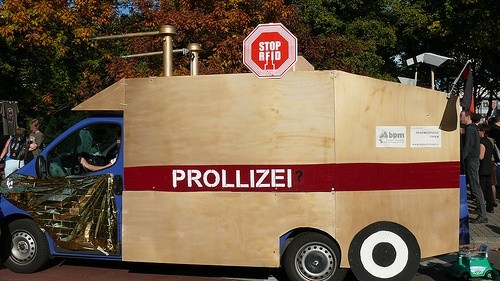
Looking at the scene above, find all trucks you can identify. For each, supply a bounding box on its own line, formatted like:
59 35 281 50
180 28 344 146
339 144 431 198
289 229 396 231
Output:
0 69 459 281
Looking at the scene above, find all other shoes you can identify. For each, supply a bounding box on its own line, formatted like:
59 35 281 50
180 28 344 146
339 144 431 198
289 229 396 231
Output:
469 200 498 214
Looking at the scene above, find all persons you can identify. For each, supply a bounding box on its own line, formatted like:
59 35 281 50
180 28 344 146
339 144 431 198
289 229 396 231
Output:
474 101 500 202
80 137 121 171
460 110 488 224
0 127 26 178
477 124 495 213
26 119 45 163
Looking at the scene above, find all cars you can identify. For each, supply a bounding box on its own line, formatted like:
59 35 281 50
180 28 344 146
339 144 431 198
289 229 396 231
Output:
447 245 496 281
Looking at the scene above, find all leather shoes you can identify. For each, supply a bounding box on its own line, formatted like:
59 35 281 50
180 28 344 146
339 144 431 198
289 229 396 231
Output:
469 214 489 224
